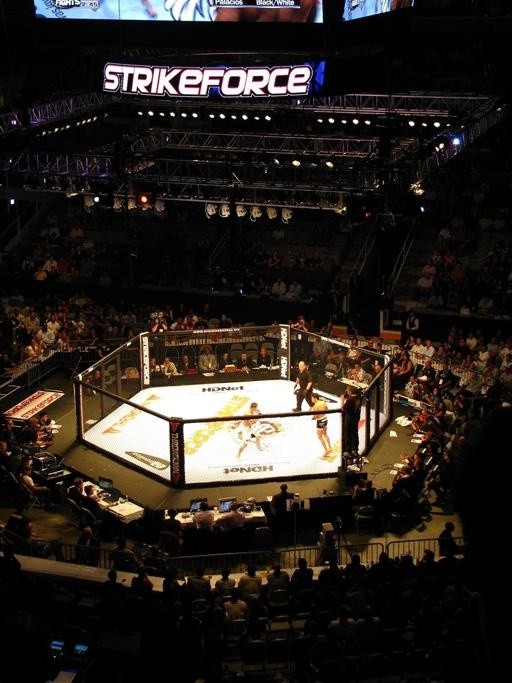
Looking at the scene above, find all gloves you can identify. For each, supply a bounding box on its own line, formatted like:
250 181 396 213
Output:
302 388 308 397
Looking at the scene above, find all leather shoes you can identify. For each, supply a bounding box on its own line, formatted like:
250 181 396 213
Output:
293 407 301 411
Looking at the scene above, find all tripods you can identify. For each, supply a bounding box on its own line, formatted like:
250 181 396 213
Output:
316 528 352 565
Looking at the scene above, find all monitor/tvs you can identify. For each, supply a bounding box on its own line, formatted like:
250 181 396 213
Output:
217 497 237 512
99 477 113 491
189 497 207 512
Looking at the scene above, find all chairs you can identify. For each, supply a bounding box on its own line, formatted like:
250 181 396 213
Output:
126 343 282 366
0 447 509 683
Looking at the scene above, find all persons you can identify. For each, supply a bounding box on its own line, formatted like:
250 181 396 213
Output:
2 0 511 681
